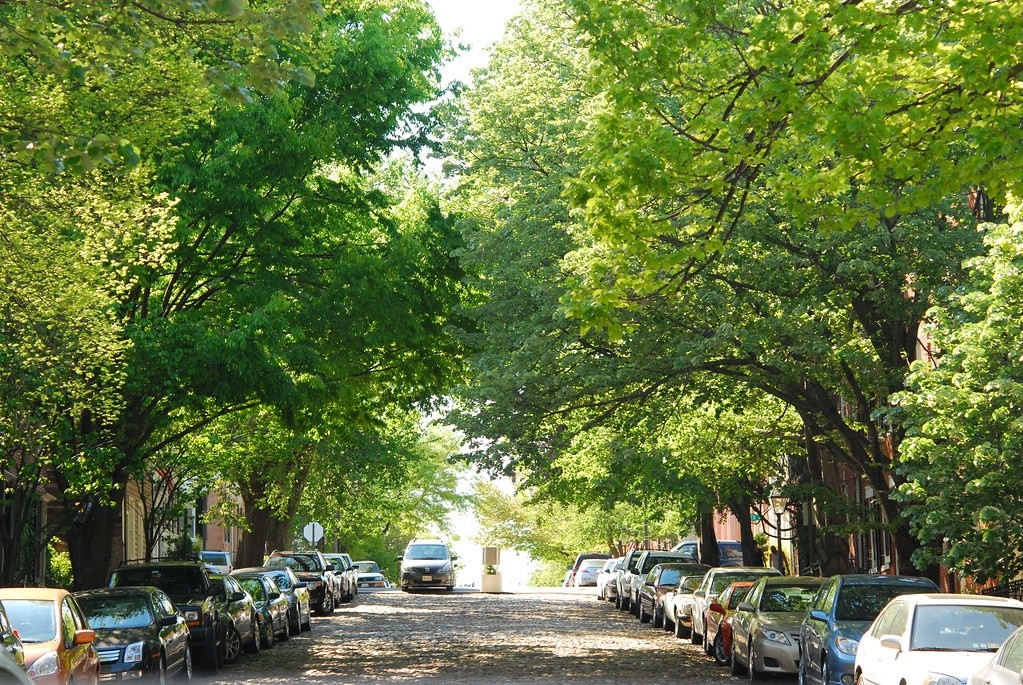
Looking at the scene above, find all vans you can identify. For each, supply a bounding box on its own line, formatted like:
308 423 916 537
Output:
670 540 746 566
200 550 234 576
396 539 457 591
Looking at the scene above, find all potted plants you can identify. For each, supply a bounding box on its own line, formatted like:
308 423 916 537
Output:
481 564 503 591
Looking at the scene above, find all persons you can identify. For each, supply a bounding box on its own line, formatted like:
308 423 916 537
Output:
753 540 767 568
769 546 790 576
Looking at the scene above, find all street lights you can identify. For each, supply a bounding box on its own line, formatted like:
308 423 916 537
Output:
63 488 95 591
770 487 788 575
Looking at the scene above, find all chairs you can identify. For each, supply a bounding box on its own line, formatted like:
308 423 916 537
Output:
412 547 425 556
367 567 374 572
965 623 984 636
788 595 803 606
434 548 445 555
919 617 942 643
767 590 786 605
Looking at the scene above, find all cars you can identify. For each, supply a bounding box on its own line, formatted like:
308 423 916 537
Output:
562 553 612 587
322 553 361 608
207 565 311 654
797 574 943 685
691 567 782 644
854 592 1023 685
596 556 625 603
730 575 830 685
354 561 393 588
705 580 756 665
636 561 712 638
0 587 193 685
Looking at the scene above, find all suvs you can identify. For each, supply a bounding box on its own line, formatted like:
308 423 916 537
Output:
107 556 221 674
262 549 335 615
616 548 672 611
